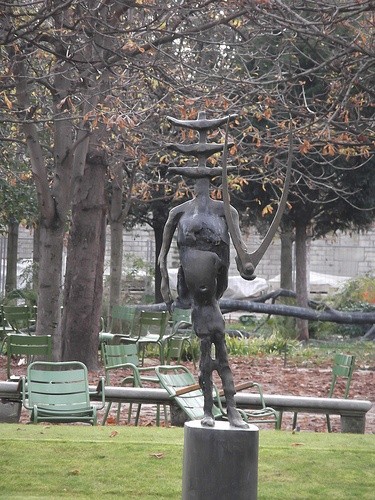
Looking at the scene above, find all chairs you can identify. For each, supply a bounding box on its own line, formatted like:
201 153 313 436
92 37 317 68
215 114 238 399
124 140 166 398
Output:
116 309 168 367
5 333 56 385
161 306 197 366
1 304 37 359
154 362 279 432
97 302 135 363
100 343 168 429
288 352 356 432
25 361 97 426
